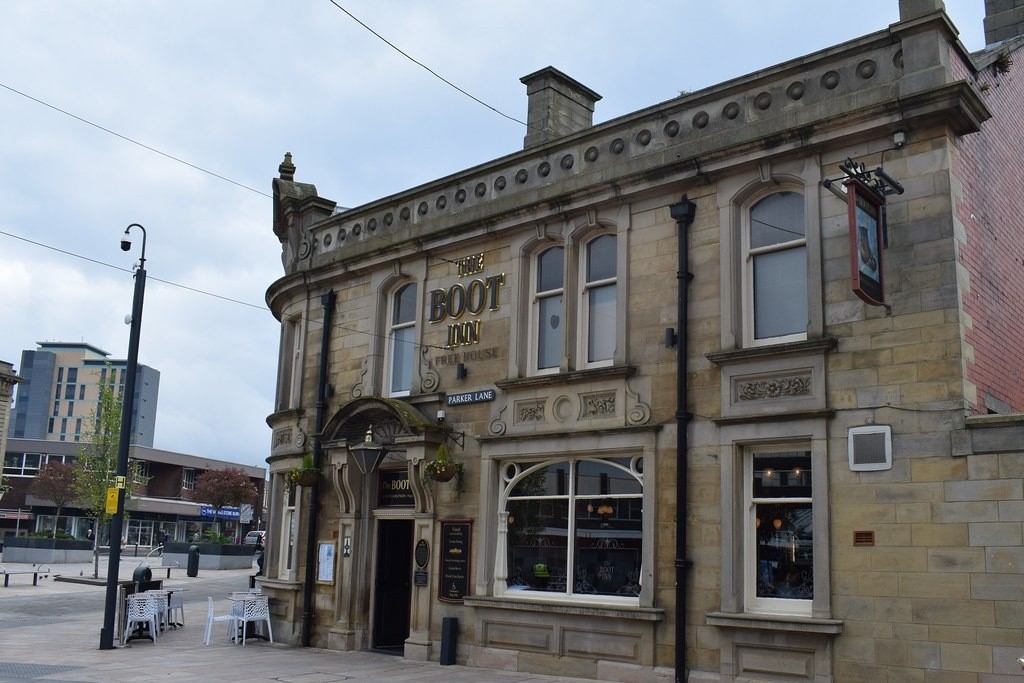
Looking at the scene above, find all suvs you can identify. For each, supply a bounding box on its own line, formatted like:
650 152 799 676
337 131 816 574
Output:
243 531 266 545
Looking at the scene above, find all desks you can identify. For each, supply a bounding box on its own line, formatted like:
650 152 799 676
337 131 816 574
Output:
226 596 270 644
162 588 189 626
125 597 163 642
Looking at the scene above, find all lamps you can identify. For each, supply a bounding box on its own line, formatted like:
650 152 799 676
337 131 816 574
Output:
324 384 334 397
456 363 467 379
665 328 674 348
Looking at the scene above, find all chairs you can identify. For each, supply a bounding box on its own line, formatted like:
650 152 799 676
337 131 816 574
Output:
204 592 273 646
123 590 185 644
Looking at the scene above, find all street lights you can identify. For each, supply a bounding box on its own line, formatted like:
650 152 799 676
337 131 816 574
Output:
97 223 146 647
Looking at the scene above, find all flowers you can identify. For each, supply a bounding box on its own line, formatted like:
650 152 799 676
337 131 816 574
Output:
421 459 466 502
289 455 312 477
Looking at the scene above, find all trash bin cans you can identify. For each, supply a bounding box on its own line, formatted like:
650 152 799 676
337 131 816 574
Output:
0 543 3 553
230 537 234 542
165 534 174 542
187 545 200 577
133 567 152 583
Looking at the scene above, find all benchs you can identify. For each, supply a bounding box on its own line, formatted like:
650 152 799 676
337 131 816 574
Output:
0 564 50 587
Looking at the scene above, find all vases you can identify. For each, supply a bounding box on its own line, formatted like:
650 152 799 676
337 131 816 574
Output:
432 465 456 482
295 472 315 487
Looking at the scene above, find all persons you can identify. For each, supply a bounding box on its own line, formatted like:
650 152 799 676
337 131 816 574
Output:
156 528 165 547
86 529 95 540
193 531 200 542
